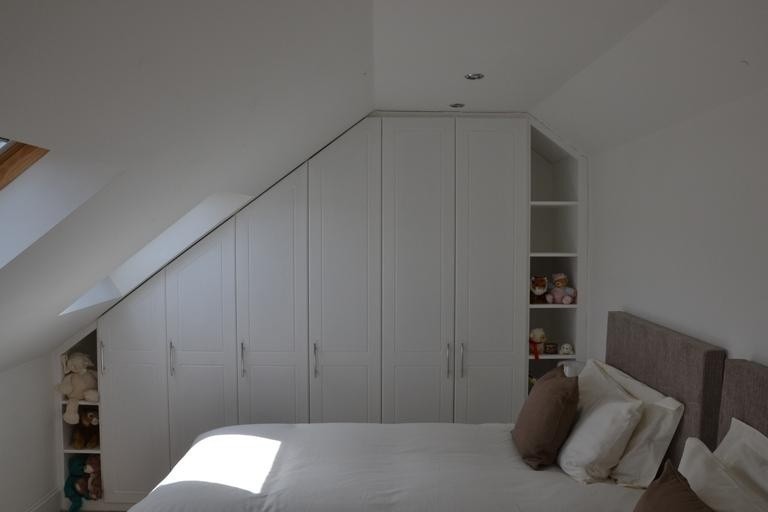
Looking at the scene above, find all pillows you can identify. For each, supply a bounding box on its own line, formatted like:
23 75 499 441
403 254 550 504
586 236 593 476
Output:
504 362 584 473
628 470 710 510
554 358 646 485
675 433 763 510
589 356 688 489
710 414 765 496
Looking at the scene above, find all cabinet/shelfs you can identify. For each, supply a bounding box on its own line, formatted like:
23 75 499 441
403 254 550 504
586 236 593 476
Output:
54 108 588 501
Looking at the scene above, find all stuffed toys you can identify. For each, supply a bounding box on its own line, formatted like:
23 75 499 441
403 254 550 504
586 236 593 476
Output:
530 324 547 356
558 340 574 355
60 351 103 508
531 270 574 304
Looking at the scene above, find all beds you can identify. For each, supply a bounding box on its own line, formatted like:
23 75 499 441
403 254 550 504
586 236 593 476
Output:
126 313 729 510
